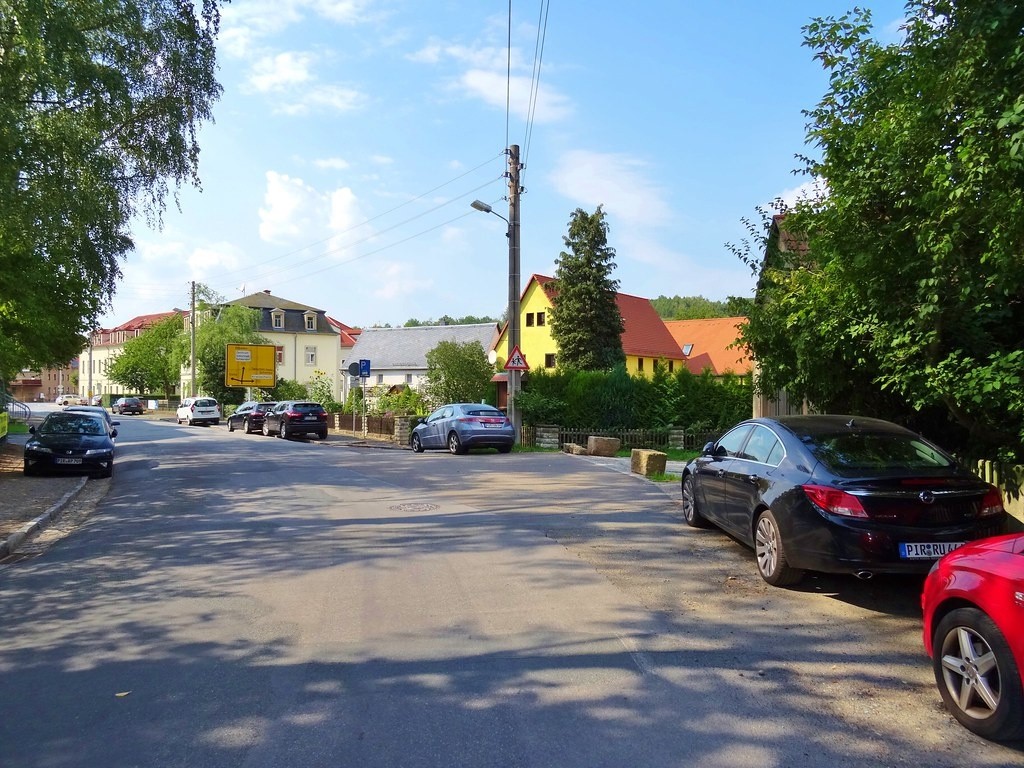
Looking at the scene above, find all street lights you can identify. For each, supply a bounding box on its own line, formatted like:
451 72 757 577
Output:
173 307 195 397
470 200 520 444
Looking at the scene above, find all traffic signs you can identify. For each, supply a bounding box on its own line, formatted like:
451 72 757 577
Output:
225 344 276 387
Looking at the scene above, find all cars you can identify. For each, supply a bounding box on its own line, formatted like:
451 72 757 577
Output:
24 412 117 476
63 406 121 443
112 398 144 415
176 397 221 426
682 414 1009 586
227 400 277 434
411 403 515 455
921 532 1024 741
56 395 101 406
262 401 328 438
